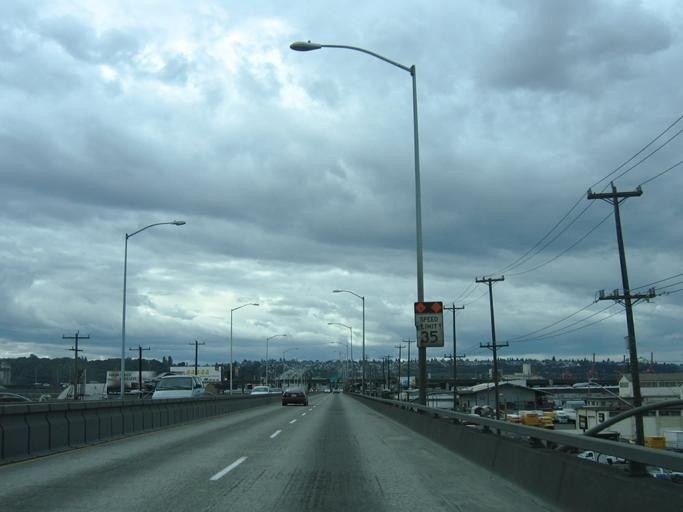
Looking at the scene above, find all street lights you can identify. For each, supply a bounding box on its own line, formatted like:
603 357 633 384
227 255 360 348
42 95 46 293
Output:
265 334 288 387
328 320 355 390
288 39 426 406
119 220 187 398
333 288 365 395
230 303 261 395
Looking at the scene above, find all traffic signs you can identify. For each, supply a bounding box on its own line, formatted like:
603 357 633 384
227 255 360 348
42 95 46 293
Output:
413 300 444 349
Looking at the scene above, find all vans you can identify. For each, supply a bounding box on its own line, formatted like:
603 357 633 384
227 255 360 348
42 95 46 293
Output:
151 374 207 399
281 388 309 406
249 386 273 394
